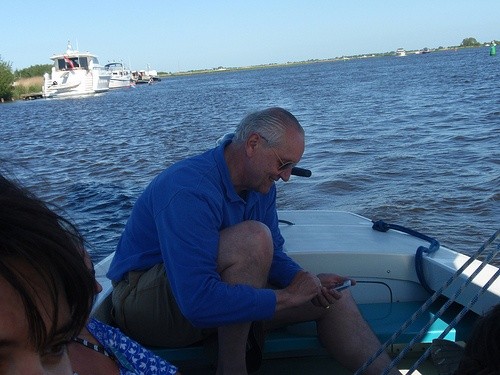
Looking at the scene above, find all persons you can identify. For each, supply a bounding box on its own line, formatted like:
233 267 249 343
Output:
0 167 179 375
106 108 403 375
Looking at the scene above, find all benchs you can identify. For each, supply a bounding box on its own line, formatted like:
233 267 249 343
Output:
152 301 473 361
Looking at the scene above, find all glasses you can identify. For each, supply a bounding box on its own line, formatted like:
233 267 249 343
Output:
258 134 297 171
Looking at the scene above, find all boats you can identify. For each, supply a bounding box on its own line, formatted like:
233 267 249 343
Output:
41 40 112 100
104 58 162 89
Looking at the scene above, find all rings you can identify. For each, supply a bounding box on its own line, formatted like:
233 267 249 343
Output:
325 305 329 309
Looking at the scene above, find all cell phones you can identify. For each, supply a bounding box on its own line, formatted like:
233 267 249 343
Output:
321 280 351 295
